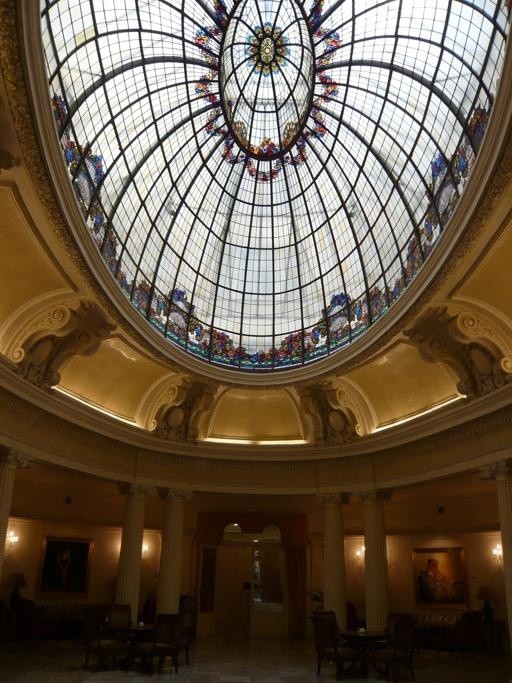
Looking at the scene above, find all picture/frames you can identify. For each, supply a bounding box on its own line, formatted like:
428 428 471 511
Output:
409 545 471 610
36 535 93 599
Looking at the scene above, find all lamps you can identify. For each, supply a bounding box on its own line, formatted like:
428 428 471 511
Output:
6 571 27 607
476 585 498 621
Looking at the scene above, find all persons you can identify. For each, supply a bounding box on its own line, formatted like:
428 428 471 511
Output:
419 557 448 601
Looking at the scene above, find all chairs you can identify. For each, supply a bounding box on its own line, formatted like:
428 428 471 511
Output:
79 601 197 674
310 609 418 681
8 594 62 657
433 611 484 664
